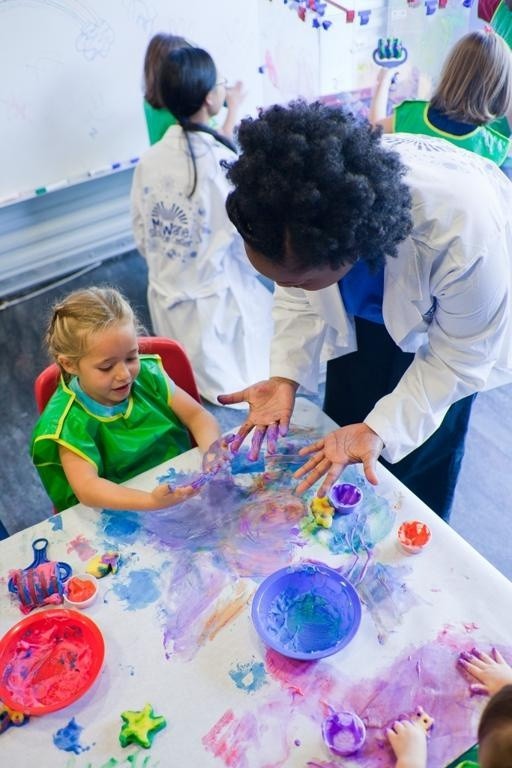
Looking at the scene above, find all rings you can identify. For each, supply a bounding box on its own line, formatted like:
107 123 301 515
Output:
266 420 279 426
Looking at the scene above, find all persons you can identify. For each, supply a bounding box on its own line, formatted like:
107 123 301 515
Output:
141 32 250 151
385 643 512 768
213 97 512 533
369 27 512 170
30 286 238 516
130 46 274 411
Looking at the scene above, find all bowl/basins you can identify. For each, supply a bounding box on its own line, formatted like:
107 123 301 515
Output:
252 564 362 663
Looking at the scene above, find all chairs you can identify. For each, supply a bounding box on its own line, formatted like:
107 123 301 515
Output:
35 338 203 449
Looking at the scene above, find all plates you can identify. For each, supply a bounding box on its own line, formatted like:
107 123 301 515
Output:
0 607 106 719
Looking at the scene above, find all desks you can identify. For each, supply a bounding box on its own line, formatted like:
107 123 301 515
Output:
0 397 512 768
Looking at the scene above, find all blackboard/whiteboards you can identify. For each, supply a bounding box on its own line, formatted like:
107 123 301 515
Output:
2 0 478 208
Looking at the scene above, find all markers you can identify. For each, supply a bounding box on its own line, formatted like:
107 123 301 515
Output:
33 178 70 195
89 162 121 177
128 156 143 165
